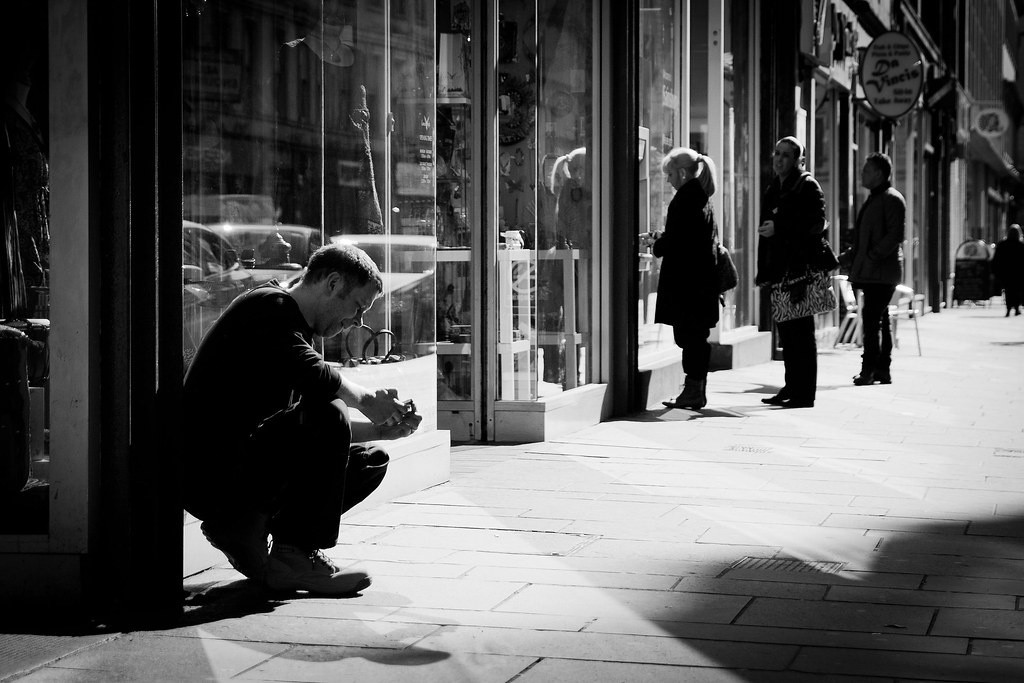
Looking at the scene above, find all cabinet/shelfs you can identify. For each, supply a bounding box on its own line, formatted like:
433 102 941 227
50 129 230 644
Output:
400 248 531 402
530 250 593 392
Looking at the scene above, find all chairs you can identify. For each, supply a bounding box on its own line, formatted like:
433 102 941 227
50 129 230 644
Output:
858 283 921 356
831 274 902 348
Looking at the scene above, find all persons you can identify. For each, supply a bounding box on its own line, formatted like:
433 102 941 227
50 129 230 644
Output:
754 136 840 409
182 243 424 594
991 224 1024 317
837 153 906 385
550 147 592 391
639 147 738 411
279 0 384 234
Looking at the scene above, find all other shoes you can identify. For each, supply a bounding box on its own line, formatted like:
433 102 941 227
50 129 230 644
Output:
762 391 818 408
854 369 893 385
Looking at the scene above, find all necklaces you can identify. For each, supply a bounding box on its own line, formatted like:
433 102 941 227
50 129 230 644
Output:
308 33 341 63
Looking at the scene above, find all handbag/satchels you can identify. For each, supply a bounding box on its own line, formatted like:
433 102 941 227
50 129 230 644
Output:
771 264 838 324
716 244 741 294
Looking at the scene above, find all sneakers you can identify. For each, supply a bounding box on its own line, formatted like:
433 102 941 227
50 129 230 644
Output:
265 534 372 597
201 519 273 584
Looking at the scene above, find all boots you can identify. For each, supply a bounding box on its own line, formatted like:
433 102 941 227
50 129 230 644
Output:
662 374 710 412
